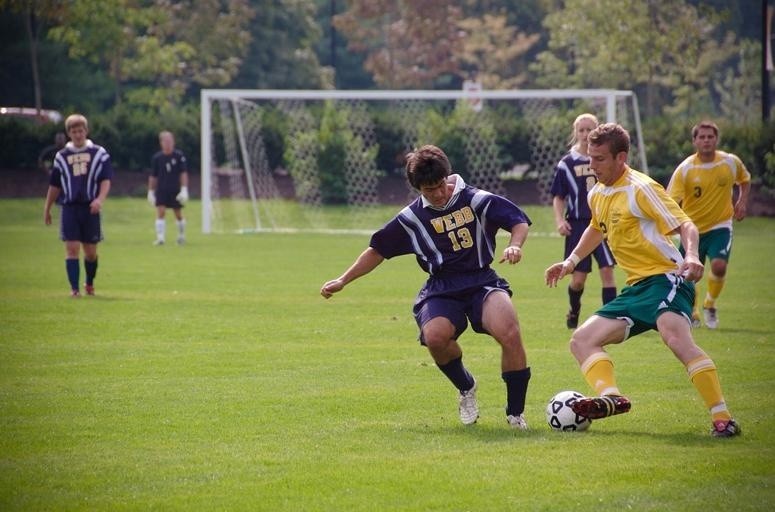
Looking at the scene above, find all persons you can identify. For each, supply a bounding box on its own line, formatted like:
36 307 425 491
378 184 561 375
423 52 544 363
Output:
314 140 535 433
665 121 755 330
553 111 618 329
545 121 742 437
38 130 67 175
40 111 114 301
142 128 192 249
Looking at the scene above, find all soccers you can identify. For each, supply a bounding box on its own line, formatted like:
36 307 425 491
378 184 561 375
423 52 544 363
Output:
545 391 592 433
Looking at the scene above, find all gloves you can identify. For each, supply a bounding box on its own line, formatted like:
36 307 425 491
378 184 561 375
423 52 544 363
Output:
148 190 157 207
176 186 189 205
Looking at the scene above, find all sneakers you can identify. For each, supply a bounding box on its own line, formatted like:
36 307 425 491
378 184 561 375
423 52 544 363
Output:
713 418 741 437
703 308 718 329
571 395 631 419
458 375 479 425
567 307 580 329
505 404 531 433
84 284 94 295
691 319 701 328
72 289 80 296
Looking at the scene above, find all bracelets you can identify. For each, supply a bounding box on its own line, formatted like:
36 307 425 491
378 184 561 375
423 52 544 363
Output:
564 252 584 266
